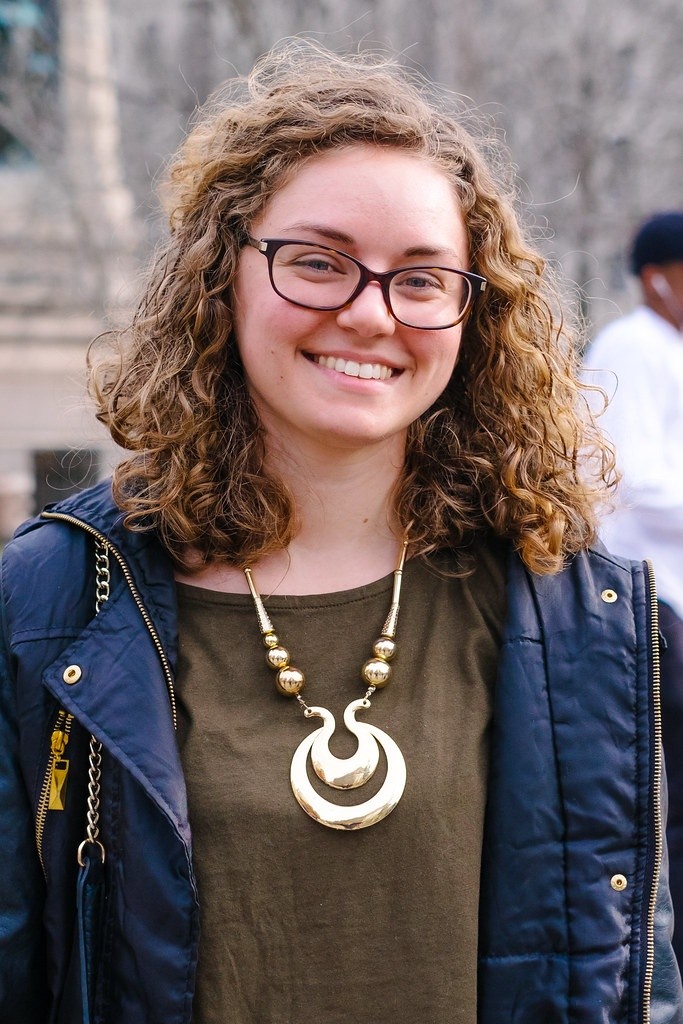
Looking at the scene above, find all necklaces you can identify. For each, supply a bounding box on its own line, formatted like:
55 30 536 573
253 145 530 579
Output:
233 501 437 834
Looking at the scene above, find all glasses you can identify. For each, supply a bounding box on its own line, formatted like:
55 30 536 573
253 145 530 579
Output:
231 233 493 332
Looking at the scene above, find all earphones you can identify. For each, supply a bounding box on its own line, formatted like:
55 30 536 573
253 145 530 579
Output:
650 274 671 297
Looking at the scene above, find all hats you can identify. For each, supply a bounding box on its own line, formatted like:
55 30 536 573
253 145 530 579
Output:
630 211 683 274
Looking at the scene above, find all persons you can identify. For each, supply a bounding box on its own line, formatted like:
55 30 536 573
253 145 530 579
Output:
567 206 683 987
0 45 683 1024
